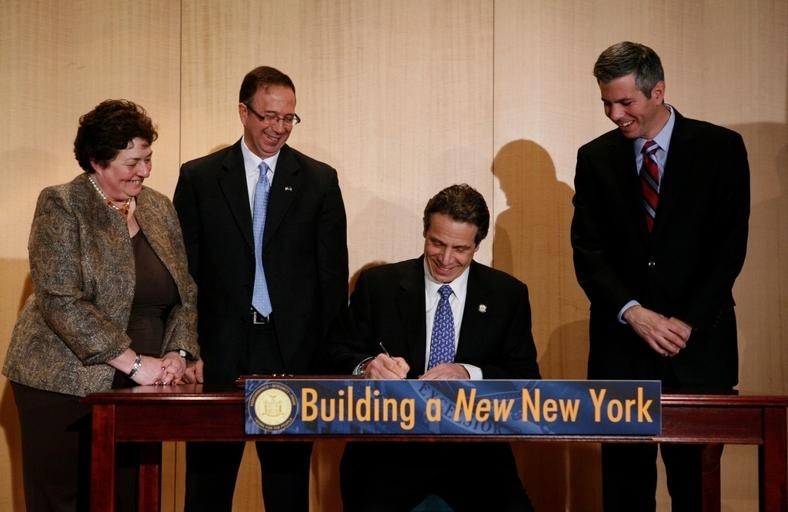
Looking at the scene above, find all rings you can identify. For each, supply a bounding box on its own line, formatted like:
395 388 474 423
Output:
379 342 392 358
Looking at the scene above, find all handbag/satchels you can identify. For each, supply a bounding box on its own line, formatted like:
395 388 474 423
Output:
357 358 373 377
128 355 142 379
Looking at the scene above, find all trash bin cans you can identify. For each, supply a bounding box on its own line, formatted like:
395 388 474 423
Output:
173 349 190 362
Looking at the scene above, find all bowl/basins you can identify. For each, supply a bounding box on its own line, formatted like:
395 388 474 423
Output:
87 176 132 220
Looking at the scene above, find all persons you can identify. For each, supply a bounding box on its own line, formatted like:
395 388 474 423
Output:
173 66 349 511
571 41 750 511
340 185 541 511
2 99 197 512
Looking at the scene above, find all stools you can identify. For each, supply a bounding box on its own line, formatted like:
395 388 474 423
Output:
250 310 275 328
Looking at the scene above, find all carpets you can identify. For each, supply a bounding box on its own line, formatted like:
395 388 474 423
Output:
428 283 456 371
639 141 662 231
249 161 277 318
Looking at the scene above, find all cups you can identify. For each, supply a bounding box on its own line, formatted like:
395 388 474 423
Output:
243 102 301 127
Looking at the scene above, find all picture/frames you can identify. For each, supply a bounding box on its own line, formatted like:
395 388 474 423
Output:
87 391 788 512
